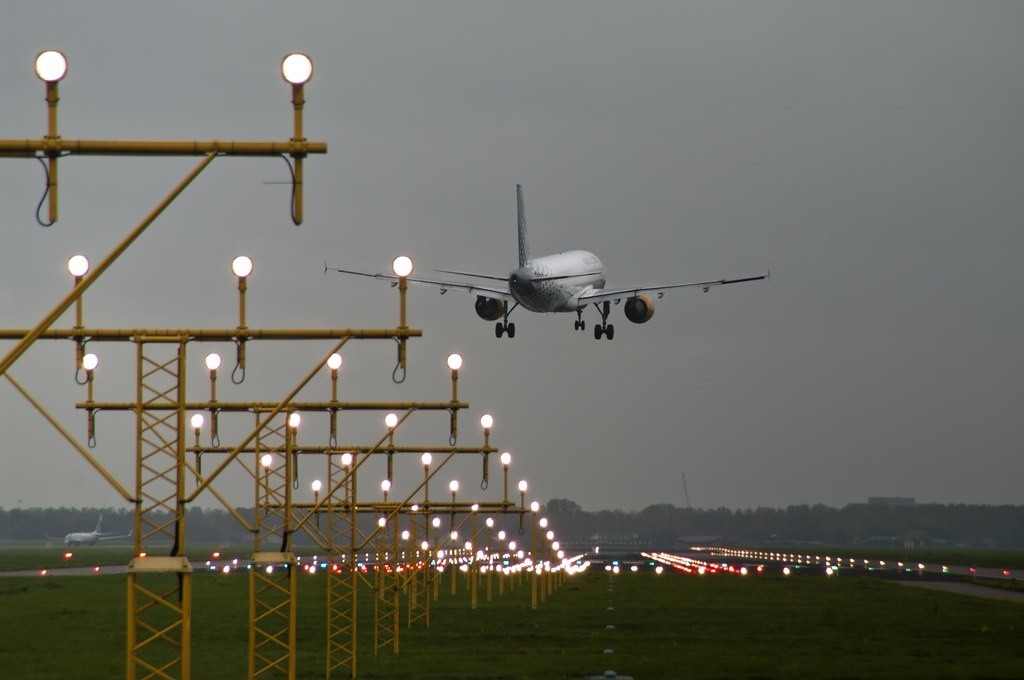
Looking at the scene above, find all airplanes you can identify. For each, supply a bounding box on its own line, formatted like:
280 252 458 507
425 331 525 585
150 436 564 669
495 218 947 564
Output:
339 183 768 340
48 514 135 547
